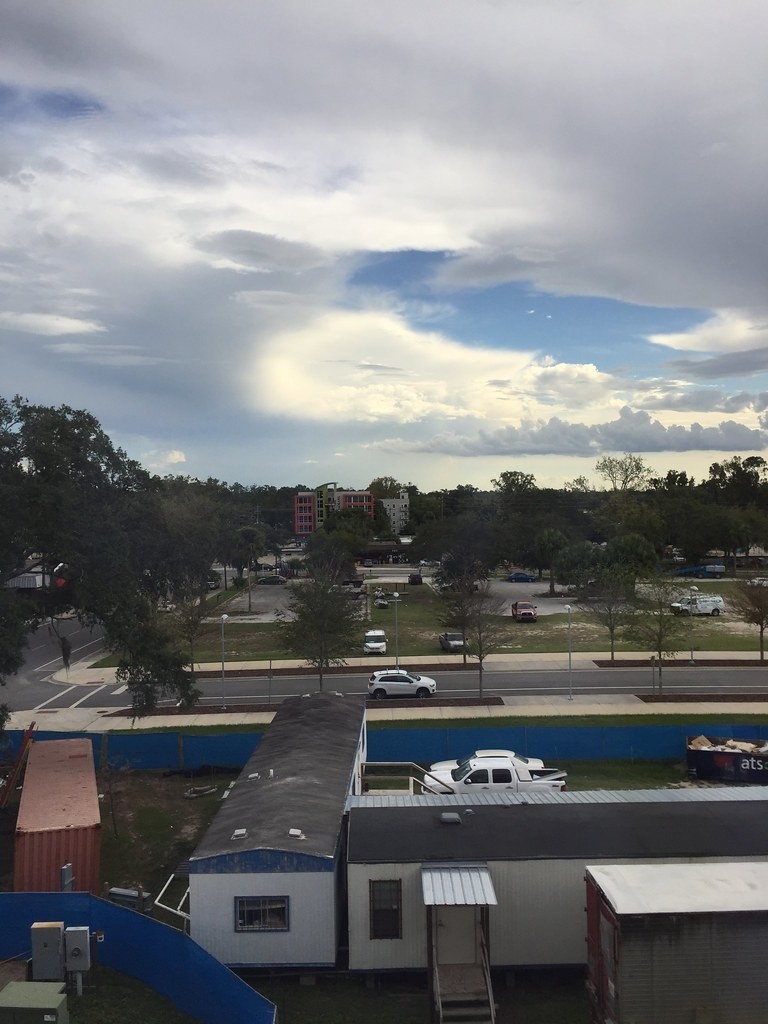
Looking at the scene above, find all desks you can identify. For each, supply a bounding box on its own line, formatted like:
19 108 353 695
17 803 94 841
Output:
386 591 410 601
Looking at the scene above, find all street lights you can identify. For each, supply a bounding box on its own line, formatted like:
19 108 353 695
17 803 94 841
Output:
393 592 400 670
690 586 698 664
564 605 574 700
221 614 228 709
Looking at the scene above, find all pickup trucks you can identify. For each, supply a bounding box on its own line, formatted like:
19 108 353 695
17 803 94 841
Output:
512 601 537 623
440 582 479 593
206 577 220 589
420 758 568 795
439 632 469 653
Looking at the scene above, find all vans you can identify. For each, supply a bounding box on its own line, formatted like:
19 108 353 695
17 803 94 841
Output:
670 593 726 617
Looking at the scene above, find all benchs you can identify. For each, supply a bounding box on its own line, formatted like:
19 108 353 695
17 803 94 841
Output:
387 600 402 603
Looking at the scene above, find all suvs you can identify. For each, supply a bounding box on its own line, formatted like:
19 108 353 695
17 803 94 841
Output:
368 670 436 698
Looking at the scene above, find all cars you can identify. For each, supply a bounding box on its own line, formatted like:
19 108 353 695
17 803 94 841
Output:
252 563 273 571
673 549 686 555
408 574 422 584
374 599 389 608
747 577 768 588
429 750 545 772
673 555 686 563
363 629 388 655
157 601 177 613
258 575 287 585
508 572 535 582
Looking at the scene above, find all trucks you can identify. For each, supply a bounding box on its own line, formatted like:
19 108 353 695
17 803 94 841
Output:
313 569 366 588
694 563 725 579
